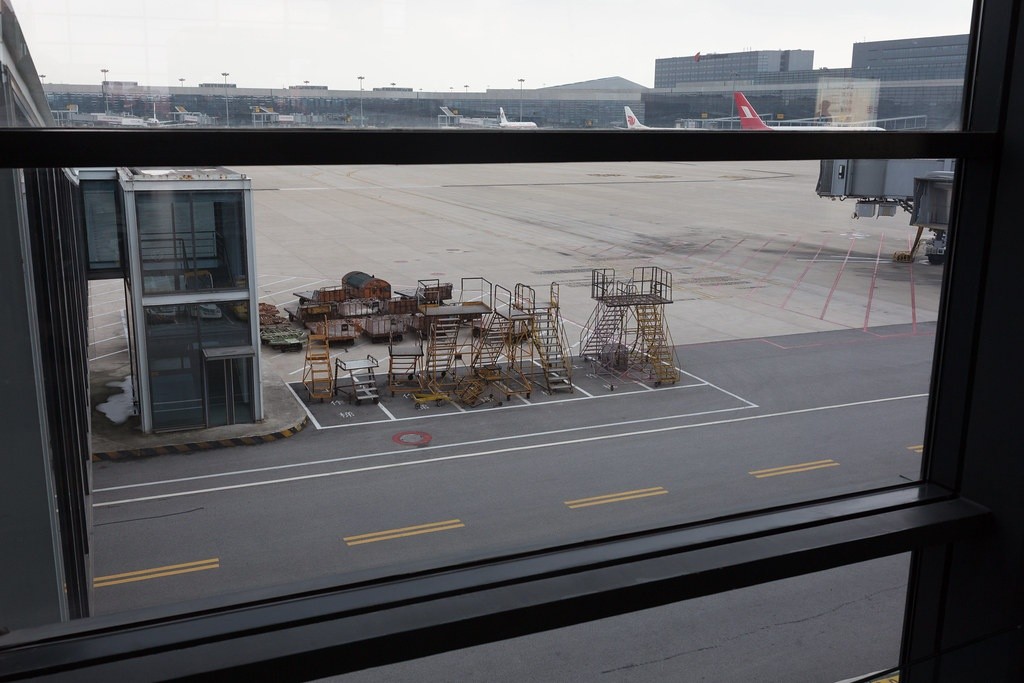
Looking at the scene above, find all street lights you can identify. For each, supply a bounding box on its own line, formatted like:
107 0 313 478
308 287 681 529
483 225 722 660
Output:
39 75 46 83
303 81 310 85
179 78 186 87
221 73 230 128
100 69 109 113
464 85 470 117
391 83 396 87
357 77 365 126
517 78 525 122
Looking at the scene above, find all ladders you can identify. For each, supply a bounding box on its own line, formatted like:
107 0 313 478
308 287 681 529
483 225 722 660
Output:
334 354 381 407
302 334 333 403
416 277 576 404
578 266 680 389
387 330 424 398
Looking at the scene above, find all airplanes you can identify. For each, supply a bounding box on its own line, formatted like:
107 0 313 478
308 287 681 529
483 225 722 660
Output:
612 106 709 130
734 91 885 132
487 107 538 130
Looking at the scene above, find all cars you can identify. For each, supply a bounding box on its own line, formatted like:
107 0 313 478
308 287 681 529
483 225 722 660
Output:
198 304 221 319
